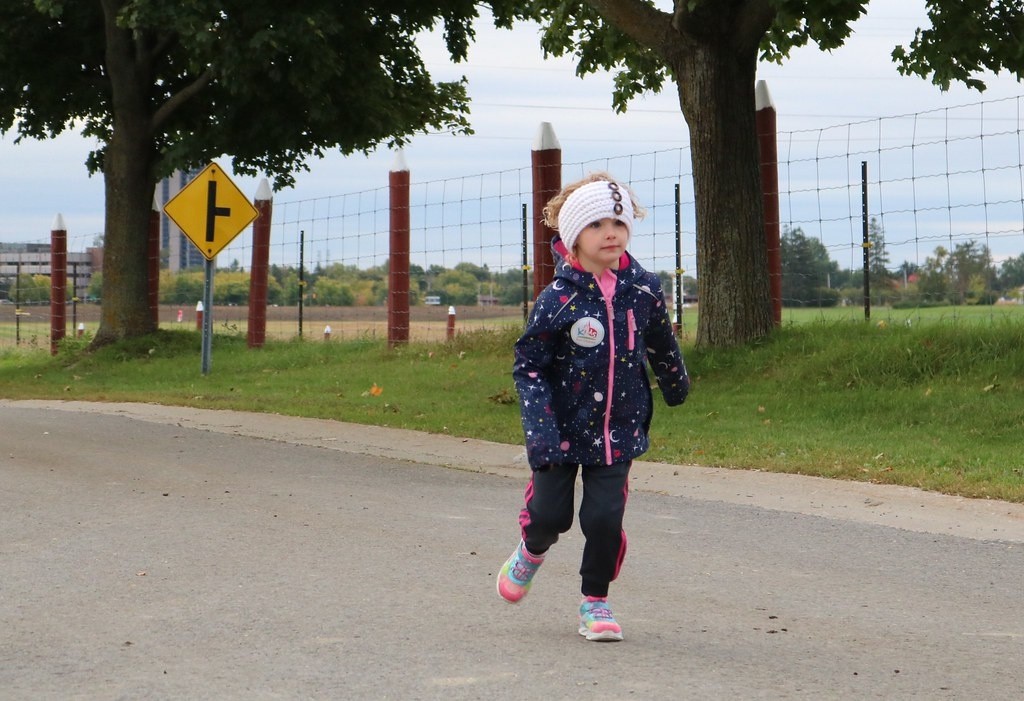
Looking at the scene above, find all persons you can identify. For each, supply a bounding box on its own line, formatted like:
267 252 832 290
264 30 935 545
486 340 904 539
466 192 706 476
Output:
494 172 690 641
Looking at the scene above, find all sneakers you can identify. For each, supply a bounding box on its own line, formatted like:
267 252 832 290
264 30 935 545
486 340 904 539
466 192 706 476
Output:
496 535 552 605
579 596 624 642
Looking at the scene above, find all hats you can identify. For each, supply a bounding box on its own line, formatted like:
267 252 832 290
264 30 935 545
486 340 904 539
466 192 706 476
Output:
557 180 633 253
